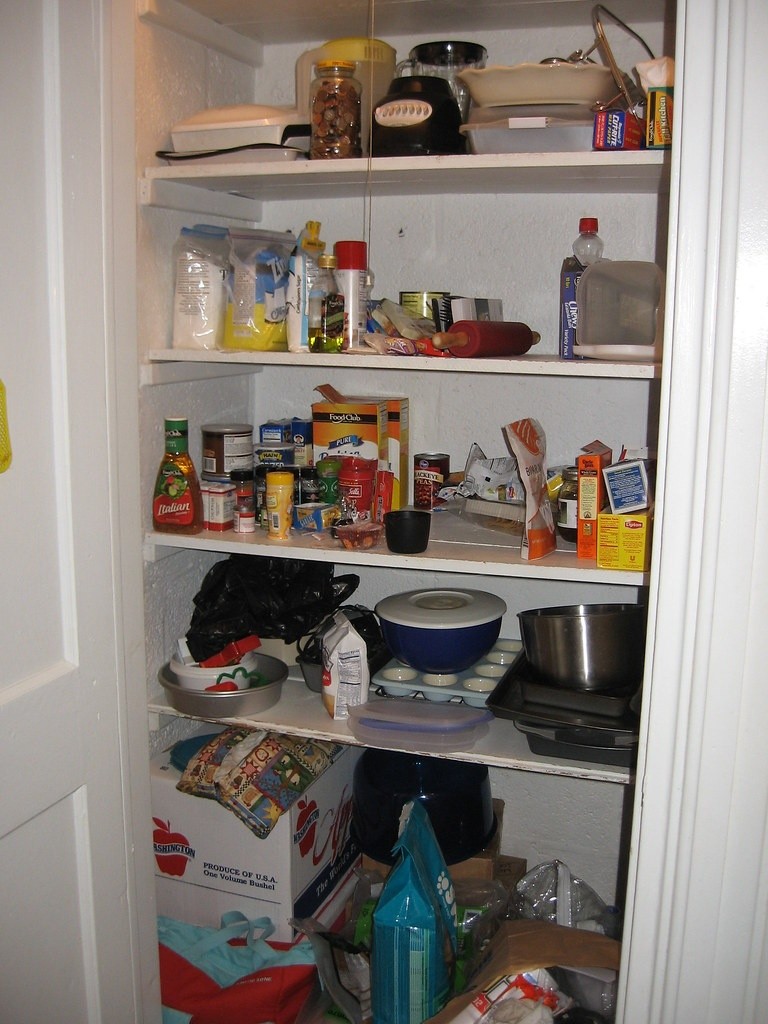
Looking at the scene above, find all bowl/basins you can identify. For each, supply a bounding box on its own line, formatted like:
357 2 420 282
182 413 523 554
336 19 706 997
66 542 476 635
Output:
170 113 307 150
375 588 507 675
515 603 647 695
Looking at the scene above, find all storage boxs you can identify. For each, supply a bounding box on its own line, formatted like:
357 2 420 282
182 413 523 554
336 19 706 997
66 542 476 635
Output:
451 299 504 323
459 106 595 154
591 109 645 150
310 383 410 519
560 255 584 360
645 87 674 150
148 724 361 944
259 417 313 466
170 113 299 161
293 503 338 532
444 855 527 963
363 798 505 887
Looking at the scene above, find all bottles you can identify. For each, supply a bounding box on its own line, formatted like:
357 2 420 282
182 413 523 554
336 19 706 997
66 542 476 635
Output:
573 217 604 268
335 239 369 352
414 452 450 509
152 416 204 535
308 255 345 352
557 466 578 542
196 422 375 540
308 60 362 159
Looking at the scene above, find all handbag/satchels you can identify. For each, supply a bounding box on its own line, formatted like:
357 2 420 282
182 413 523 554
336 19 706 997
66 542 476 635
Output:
153 910 318 1024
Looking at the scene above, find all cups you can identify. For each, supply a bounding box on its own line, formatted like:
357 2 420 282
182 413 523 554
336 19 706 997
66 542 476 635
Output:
385 511 430 552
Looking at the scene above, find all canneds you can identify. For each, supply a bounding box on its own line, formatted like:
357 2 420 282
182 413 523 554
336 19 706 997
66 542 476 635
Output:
414 453 450 511
200 423 341 535
399 291 451 323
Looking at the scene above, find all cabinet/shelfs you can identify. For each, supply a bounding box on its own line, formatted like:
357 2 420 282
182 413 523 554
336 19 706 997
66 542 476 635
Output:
91 1 685 1024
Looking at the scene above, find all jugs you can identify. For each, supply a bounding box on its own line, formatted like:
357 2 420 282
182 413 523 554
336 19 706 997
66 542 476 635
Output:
295 36 396 140
397 41 489 125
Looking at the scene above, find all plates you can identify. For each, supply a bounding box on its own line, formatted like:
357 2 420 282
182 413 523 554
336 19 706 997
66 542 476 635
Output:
456 64 641 106
164 148 300 167
